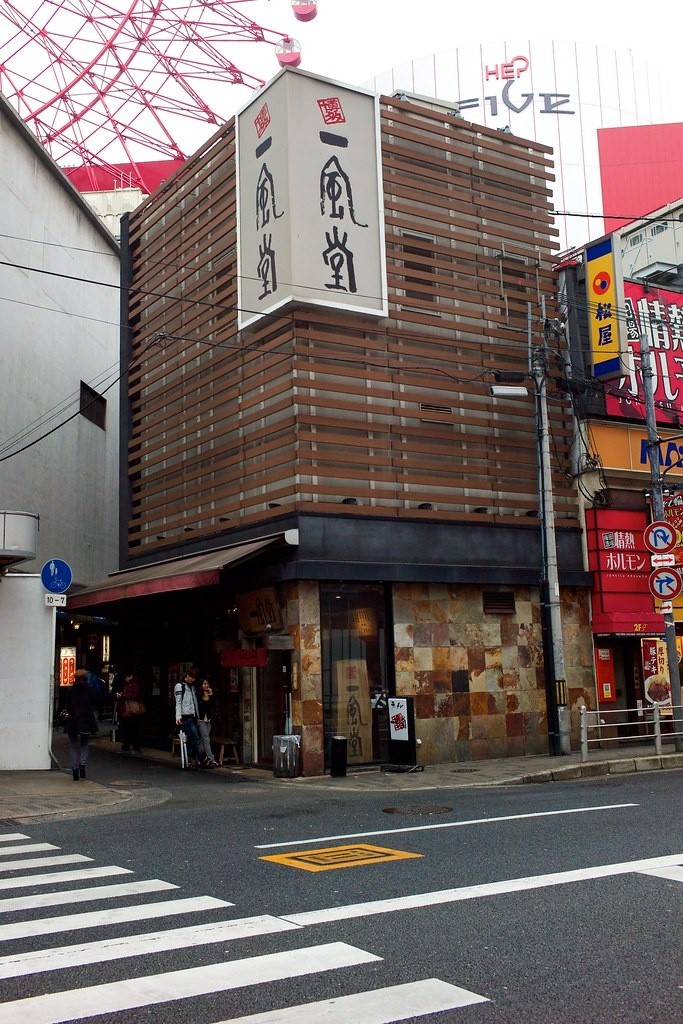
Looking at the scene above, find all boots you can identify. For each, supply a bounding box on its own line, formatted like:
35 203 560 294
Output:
79 764 86 778
73 768 79 780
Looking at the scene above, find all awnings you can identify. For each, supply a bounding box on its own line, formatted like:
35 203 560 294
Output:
57 535 294 613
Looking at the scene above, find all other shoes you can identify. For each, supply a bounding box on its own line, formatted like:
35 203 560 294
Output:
116 748 130 754
132 750 139 755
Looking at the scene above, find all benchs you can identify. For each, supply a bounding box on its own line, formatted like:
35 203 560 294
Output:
208 736 240 766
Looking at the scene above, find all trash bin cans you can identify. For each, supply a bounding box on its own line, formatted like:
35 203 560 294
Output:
272 735 301 777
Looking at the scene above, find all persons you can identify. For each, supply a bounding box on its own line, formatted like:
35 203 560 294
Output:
111 666 145 754
63 669 109 781
174 667 214 769
196 678 218 765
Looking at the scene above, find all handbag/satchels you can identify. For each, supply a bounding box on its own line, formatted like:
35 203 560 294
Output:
126 701 146 716
